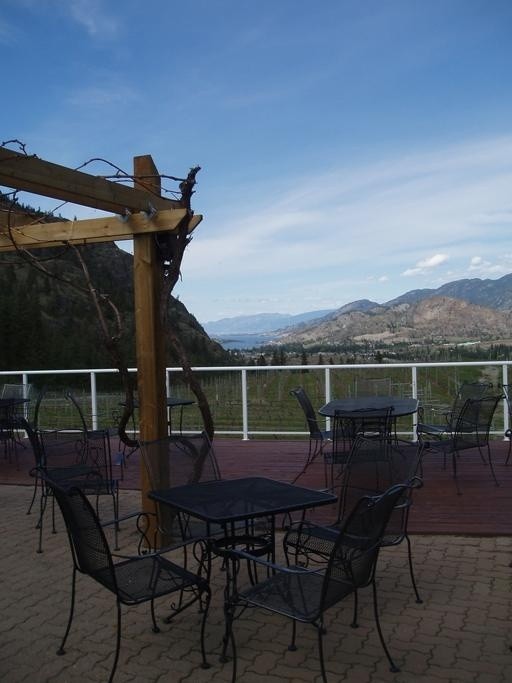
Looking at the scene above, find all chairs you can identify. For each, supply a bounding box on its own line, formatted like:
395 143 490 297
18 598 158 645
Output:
62 389 126 466
289 380 505 487
4 382 53 468
31 468 423 683
26 429 121 554
